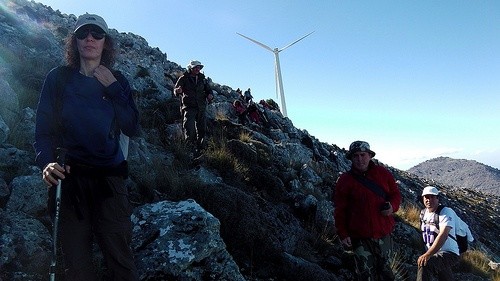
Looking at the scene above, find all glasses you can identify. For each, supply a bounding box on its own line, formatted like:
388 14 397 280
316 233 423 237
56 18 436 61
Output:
75 25 105 40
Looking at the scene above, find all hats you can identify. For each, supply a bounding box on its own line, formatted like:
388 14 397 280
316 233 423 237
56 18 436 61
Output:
73 13 109 35
345 140 376 160
420 186 439 197
187 60 204 71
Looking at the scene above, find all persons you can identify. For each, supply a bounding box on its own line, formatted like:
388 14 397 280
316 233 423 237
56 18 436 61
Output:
244 88 252 103
247 100 259 123
233 101 249 118
33 14 140 281
416 186 460 281
173 60 214 150
333 141 401 281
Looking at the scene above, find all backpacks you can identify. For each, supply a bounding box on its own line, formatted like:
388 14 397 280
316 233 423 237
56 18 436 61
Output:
433 205 470 254
